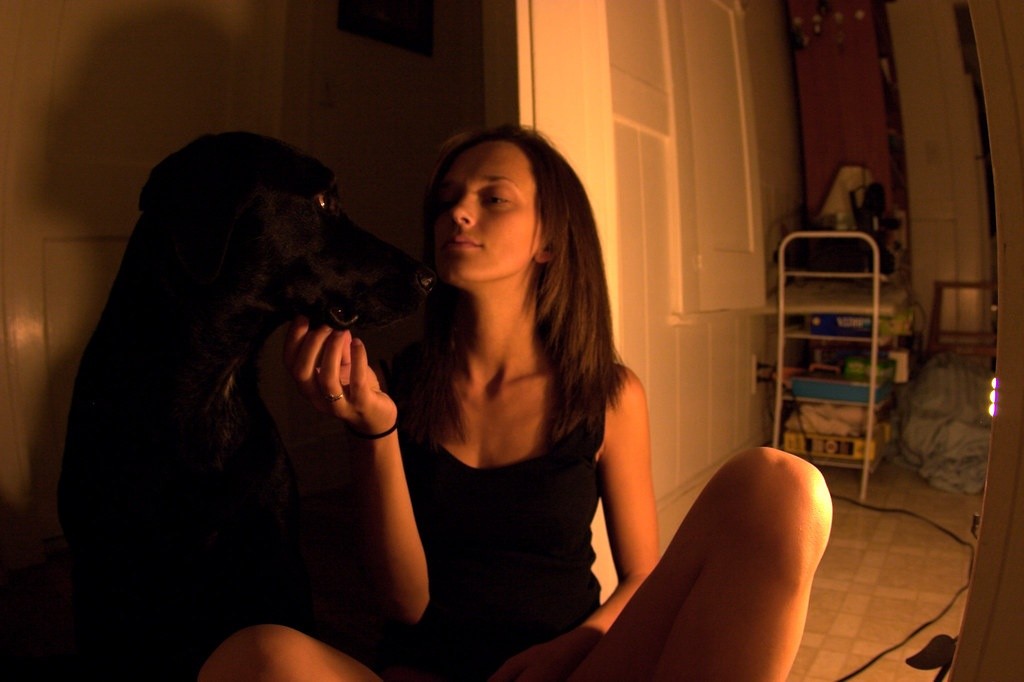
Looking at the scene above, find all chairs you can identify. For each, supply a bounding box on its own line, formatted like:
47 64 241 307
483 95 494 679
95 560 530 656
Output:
929 280 997 363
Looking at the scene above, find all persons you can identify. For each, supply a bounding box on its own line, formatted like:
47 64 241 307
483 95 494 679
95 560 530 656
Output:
197 124 834 682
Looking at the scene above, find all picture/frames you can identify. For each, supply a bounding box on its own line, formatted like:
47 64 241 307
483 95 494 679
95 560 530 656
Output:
336 0 434 58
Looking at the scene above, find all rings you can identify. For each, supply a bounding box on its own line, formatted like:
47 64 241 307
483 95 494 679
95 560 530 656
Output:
325 393 342 402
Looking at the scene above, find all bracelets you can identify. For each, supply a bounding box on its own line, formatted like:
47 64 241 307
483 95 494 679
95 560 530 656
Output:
344 420 398 440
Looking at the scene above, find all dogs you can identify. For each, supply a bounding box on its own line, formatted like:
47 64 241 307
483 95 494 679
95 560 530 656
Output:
53 131 436 682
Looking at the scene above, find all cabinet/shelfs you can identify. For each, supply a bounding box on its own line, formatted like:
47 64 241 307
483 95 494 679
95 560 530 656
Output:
755 232 913 499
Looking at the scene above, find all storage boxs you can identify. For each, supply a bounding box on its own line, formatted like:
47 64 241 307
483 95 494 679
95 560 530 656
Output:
816 348 912 383
879 421 898 443
791 375 893 403
783 427 886 461
846 358 898 382
809 314 874 338
